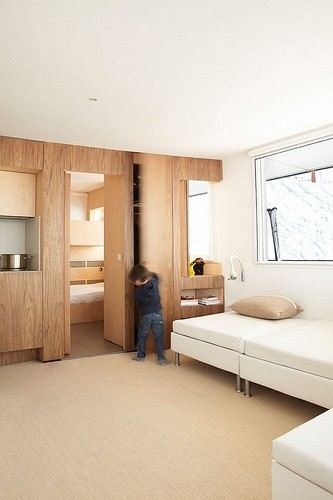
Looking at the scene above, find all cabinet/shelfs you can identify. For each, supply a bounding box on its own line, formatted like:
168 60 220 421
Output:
181 276 224 319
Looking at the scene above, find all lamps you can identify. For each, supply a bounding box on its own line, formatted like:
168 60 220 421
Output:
230 257 245 283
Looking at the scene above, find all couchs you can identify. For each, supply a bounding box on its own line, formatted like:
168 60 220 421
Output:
170 265 333 409
271 410 333 499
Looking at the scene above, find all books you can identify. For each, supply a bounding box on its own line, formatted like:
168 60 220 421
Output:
180 295 221 307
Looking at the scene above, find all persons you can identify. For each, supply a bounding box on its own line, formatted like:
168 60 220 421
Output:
128 265 169 365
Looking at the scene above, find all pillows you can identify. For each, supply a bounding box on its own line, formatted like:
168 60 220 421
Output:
229 296 306 321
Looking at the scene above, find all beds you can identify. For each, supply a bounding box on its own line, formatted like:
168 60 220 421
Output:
71 282 104 324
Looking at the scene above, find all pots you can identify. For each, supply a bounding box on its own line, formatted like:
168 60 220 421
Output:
0 254 33 269
